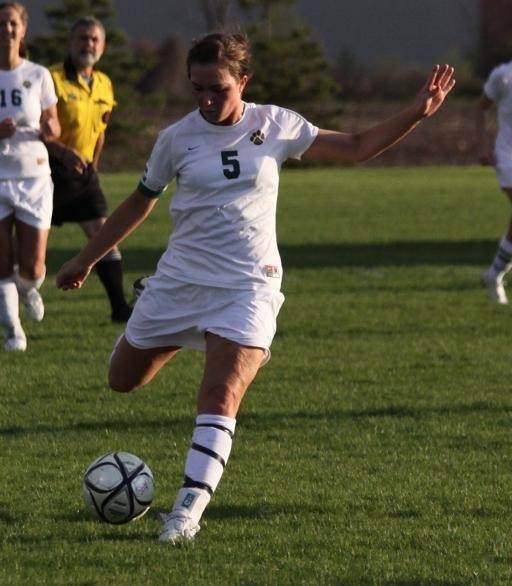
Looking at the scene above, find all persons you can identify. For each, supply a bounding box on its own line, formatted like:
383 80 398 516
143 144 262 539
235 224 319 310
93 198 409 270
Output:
477 58 512 304
0 0 61 352
46 15 142 323
59 32 456 545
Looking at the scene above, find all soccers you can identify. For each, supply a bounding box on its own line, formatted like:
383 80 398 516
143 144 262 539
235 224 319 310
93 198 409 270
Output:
81 450 155 523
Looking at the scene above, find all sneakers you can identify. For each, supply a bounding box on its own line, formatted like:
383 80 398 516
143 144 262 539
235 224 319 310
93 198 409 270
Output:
23 290 46 321
157 507 199 547
479 268 507 306
4 327 26 352
